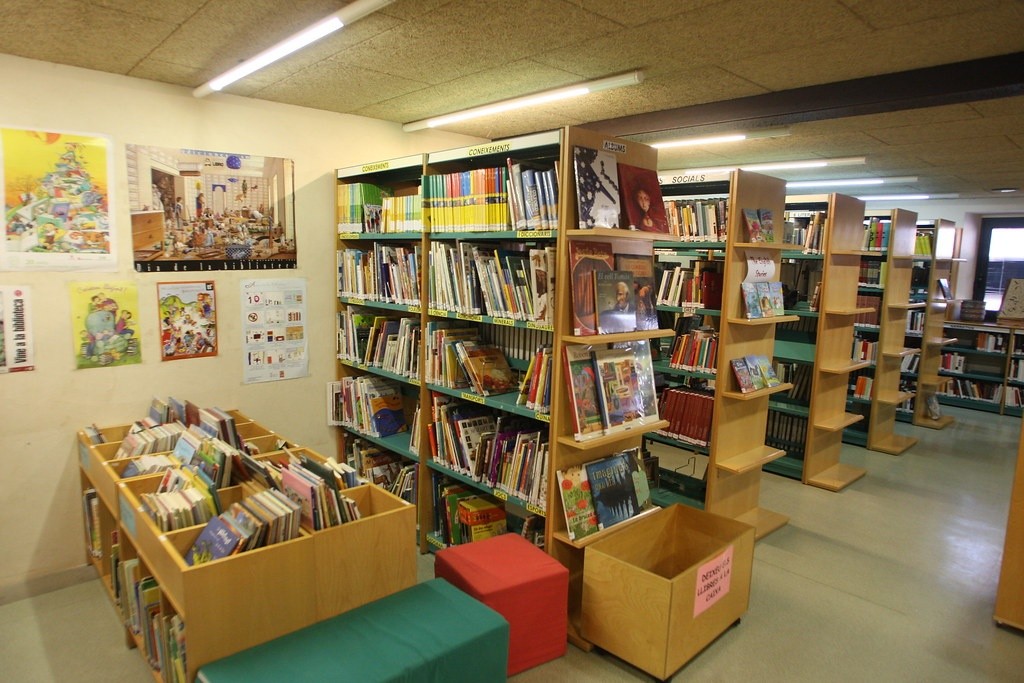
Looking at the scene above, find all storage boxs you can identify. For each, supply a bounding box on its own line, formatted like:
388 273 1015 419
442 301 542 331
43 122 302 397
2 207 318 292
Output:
578 501 758 683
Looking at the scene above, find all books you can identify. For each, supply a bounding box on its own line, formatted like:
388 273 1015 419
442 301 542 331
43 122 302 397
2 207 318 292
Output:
83 395 359 683
423 322 555 416
326 375 420 456
555 145 1024 542
337 242 422 309
337 183 422 234
336 305 421 381
426 391 547 548
429 238 558 326
343 435 420 526
422 157 560 233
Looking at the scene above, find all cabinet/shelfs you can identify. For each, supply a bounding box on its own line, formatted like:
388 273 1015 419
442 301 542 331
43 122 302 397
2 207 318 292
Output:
420 126 564 555
334 152 425 544
78 417 214 651
911 219 968 430
231 445 415 625
119 461 319 682
804 192 882 493
866 206 931 457
180 409 299 460
706 168 806 542
841 206 895 448
896 218 936 422
633 170 733 508
763 192 834 484
548 124 682 653
932 319 1024 417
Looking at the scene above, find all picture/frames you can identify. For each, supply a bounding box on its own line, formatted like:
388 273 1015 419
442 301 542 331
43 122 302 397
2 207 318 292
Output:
0 123 118 273
997 278 1024 329
155 279 218 361
